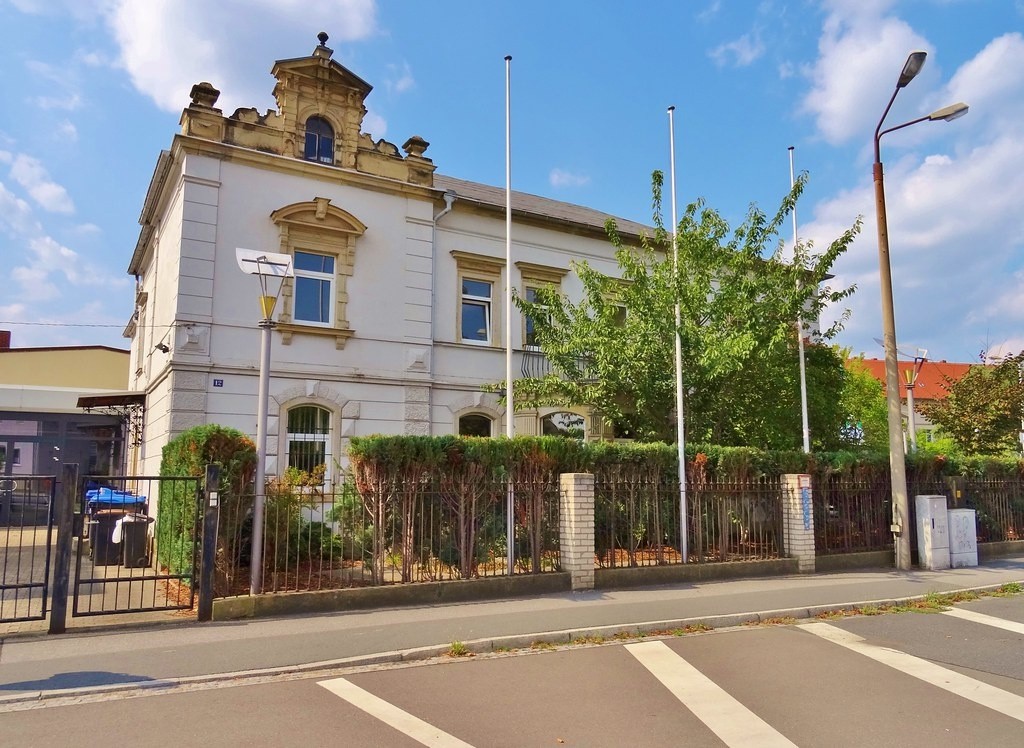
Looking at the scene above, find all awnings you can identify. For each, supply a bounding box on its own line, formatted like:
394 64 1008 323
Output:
75 393 147 446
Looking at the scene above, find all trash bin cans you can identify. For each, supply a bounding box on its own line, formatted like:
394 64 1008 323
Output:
93 508 130 567
86 486 146 560
123 511 155 569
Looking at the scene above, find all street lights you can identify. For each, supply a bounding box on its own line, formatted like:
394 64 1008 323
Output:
872 51 970 572
